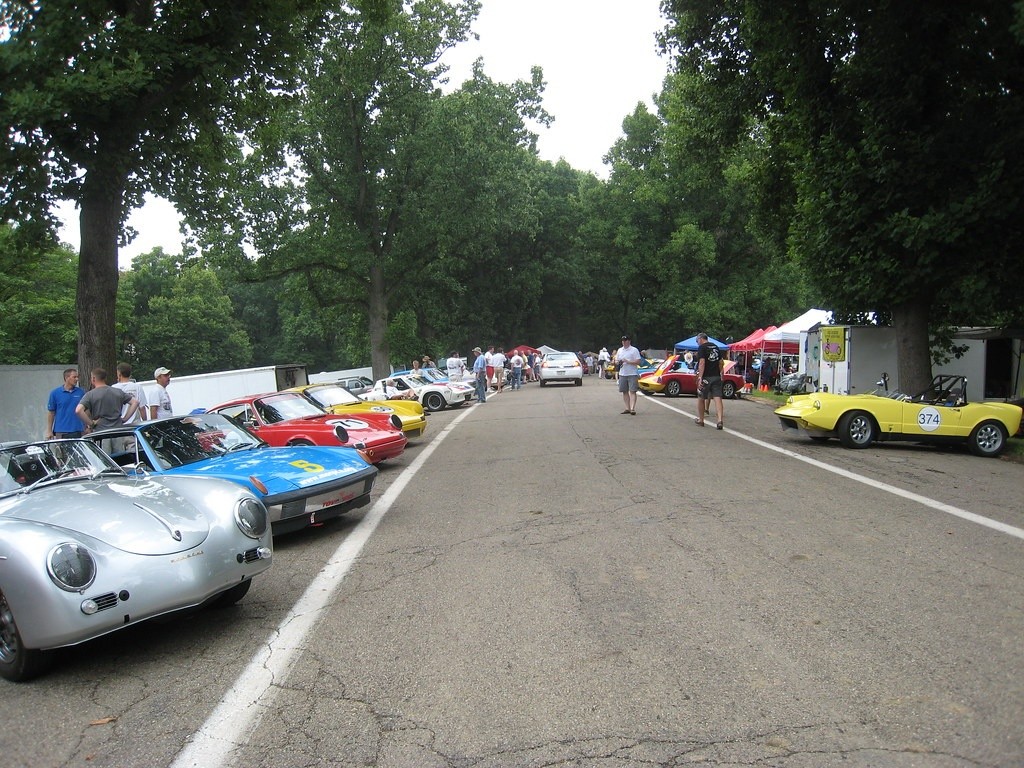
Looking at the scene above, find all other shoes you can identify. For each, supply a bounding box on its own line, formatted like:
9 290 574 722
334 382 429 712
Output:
716 421 723 429
695 419 704 426
630 410 637 415
621 410 630 414
475 399 486 403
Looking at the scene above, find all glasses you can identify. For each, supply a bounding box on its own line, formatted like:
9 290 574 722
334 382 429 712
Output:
696 337 702 343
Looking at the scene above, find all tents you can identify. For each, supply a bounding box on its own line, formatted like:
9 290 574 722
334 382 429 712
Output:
727 308 1024 404
507 345 559 358
674 335 729 361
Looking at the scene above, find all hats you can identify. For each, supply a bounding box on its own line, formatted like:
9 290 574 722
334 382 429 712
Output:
472 346 482 353
153 366 172 379
620 334 633 341
422 356 431 362
601 347 607 352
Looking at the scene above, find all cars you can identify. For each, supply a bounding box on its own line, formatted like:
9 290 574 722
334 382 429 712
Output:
535 352 583 387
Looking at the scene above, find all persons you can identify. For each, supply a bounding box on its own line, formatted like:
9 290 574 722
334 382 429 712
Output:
485 345 540 393
386 379 431 416
695 333 724 429
584 347 616 379
148 367 173 420
110 363 147 453
615 335 641 415
470 347 485 403
76 368 140 456
684 349 693 367
446 351 465 377
45 369 86 440
410 360 423 375
422 355 436 368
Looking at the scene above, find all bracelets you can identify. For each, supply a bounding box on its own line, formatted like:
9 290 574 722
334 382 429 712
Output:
89 421 94 427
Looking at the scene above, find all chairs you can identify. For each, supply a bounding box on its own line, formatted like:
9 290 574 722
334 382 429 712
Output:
943 388 961 406
925 384 936 400
8 450 50 485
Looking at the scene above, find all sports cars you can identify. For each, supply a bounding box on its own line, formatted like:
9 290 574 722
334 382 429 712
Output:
333 367 510 411
282 382 427 441
773 370 1024 458
634 355 744 397
184 390 408 468
62 413 379 538
0 436 275 684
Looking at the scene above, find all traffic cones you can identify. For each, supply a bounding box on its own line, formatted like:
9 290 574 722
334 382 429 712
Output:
760 385 765 391
764 384 769 392
